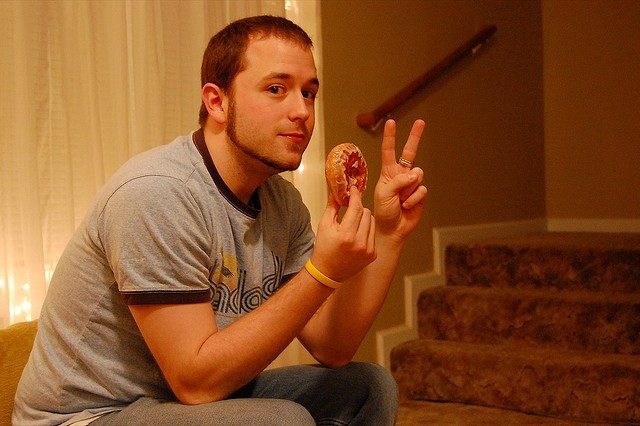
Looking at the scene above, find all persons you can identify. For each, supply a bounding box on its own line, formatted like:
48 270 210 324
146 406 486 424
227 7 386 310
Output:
10 14 428 426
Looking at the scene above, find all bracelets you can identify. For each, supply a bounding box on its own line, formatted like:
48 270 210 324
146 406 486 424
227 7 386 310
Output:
304 258 343 290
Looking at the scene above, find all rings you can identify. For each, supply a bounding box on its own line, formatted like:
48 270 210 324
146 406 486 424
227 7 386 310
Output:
397 157 415 169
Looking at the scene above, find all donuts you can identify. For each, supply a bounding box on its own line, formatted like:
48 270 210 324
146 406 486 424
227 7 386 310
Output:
325 142 368 208
